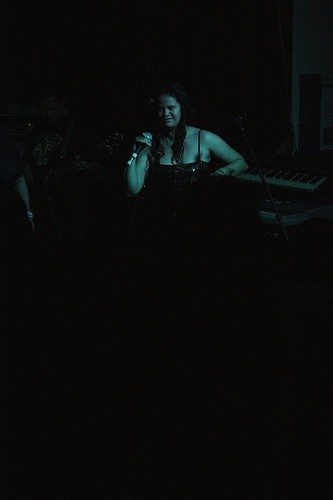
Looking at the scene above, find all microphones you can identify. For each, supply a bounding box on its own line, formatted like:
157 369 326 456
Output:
128 132 153 165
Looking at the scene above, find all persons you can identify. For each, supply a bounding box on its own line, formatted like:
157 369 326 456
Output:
0 137 37 231
126 82 248 195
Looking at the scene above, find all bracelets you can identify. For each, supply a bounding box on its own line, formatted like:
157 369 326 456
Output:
27 209 33 220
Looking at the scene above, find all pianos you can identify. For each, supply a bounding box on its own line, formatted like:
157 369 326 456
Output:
238 163 333 205
